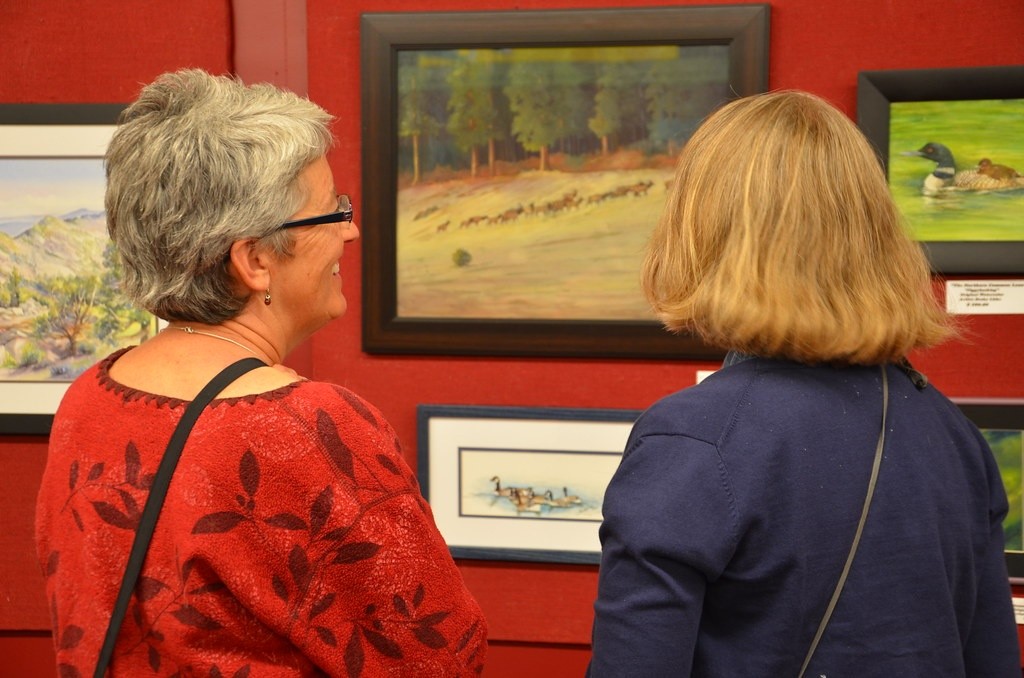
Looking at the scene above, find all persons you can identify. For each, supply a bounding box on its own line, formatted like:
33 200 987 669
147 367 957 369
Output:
35 66 490 678
581 90 1024 678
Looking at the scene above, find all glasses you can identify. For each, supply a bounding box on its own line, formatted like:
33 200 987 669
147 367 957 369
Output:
276 195 353 232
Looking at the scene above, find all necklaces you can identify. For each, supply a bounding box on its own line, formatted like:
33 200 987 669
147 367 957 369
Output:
158 325 261 359
